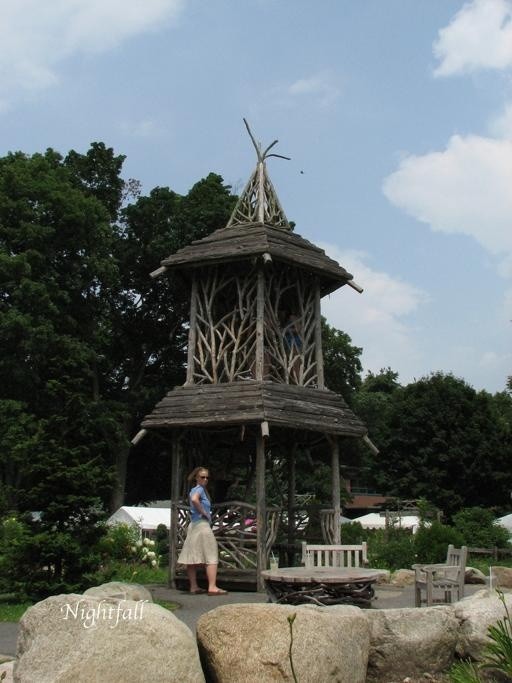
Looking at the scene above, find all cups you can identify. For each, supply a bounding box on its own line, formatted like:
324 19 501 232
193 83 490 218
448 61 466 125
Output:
268 557 281 576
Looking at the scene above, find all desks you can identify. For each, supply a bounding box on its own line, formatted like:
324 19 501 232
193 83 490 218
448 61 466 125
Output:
260 567 390 606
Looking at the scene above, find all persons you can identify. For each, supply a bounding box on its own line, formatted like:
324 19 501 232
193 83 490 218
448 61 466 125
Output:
177 467 229 595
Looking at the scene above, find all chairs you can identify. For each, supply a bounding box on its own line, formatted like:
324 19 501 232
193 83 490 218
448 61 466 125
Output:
412 545 467 608
301 542 369 567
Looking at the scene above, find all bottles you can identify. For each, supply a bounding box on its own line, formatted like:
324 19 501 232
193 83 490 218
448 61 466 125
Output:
304 547 313 570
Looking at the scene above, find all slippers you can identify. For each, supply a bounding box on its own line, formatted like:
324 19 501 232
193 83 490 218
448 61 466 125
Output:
207 590 226 595
191 589 206 594
